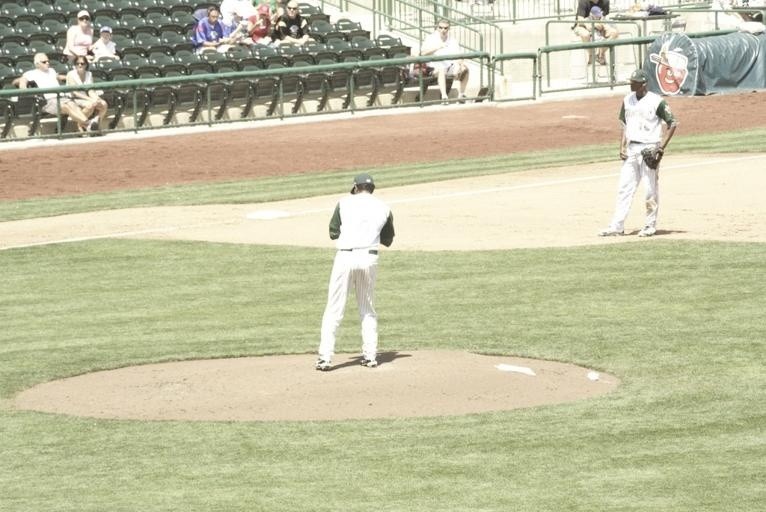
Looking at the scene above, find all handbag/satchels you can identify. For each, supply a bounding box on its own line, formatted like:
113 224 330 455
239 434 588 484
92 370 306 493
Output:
402 56 429 81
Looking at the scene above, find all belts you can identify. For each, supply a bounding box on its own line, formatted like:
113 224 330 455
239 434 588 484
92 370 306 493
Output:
340 249 377 254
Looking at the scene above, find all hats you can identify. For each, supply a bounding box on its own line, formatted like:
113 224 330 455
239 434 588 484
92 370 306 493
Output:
77 9 91 19
625 69 649 83
350 173 375 194
258 5 269 16
100 26 113 34
590 6 602 18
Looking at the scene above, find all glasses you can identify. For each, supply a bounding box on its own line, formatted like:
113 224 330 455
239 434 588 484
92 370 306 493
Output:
76 62 85 65
79 17 89 21
41 60 49 63
288 7 297 10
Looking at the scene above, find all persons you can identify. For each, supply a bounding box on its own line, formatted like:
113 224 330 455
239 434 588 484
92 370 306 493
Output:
584 6 607 62
420 19 470 105
598 70 677 238
315 173 395 371
571 0 618 64
12 9 122 137
193 0 316 56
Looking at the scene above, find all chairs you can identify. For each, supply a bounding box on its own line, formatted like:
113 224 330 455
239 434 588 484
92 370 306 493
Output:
0 0 464 139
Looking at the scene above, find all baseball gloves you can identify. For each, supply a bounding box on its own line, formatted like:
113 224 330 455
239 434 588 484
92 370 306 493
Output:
641 144 664 169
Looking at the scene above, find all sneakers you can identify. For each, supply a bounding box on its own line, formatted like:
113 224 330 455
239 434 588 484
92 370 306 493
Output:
637 225 657 237
78 115 105 137
359 359 377 368
595 54 606 65
598 227 624 236
457 94 466 104
313 358 334 371
440 98 448 105
